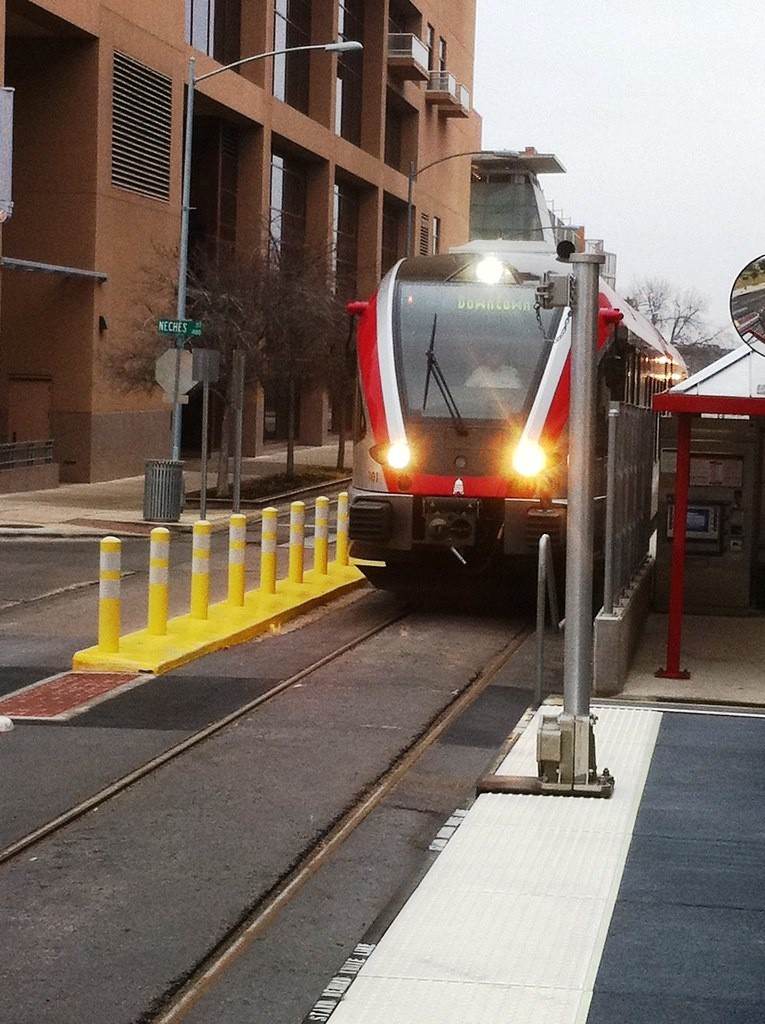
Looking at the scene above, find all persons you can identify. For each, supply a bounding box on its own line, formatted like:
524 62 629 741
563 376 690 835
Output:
466 344 523 388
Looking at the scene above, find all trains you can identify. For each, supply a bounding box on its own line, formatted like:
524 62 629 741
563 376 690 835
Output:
346 239 690 617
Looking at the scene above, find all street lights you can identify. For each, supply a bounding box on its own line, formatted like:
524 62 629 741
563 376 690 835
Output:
404 150 520 257
170 40 363 514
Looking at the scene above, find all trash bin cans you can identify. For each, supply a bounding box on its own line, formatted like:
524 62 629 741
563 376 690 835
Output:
144 459 185 523
